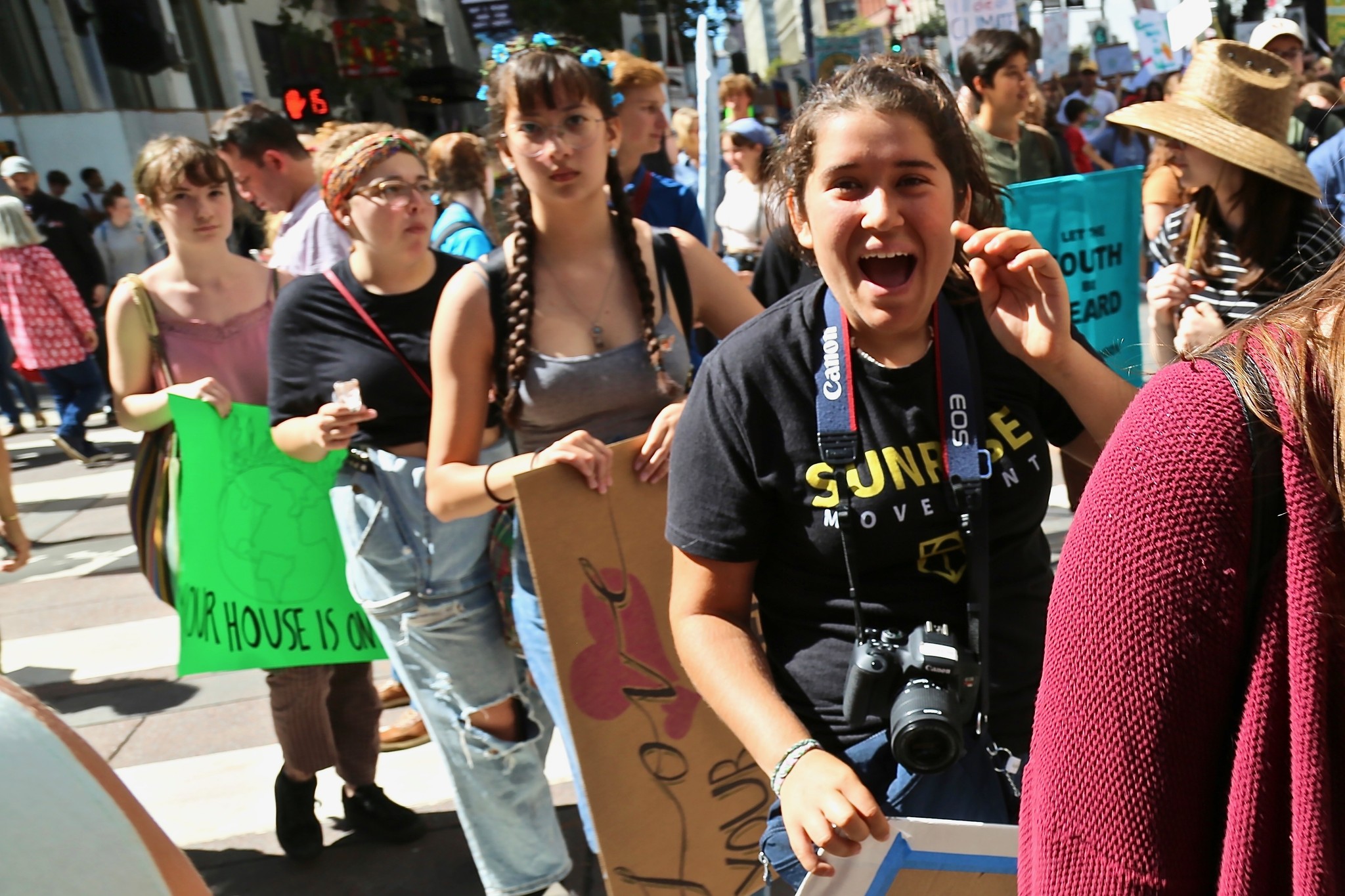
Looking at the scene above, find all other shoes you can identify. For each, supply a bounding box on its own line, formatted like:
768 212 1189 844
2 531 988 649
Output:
376 672 410 708
341 782 424 842
33 412 46 427
4 424 26 437
84 448 113 463
53 425 89 463
274 766 323 863
379 710 432 752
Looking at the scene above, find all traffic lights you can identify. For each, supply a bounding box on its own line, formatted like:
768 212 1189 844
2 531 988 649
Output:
892 37 901 56
90 0 166 78
282 83 330 123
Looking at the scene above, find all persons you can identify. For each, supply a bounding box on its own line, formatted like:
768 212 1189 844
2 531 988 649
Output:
1 1 1345 896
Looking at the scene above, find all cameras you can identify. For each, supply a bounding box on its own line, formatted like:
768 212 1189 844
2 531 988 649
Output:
843 616 991 776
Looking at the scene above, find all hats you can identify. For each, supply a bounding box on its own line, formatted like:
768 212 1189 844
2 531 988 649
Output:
1 155 34 177
727 117 768 140
1248 19 1308 50
1105 38 1321 200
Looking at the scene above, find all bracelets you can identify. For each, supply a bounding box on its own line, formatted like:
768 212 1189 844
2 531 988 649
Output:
771 738 823 799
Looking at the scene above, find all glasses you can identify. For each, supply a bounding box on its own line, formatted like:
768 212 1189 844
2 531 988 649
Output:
347 178 446 213
501 114 615 158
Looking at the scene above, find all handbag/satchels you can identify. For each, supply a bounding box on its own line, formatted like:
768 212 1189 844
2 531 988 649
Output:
126 422 179 610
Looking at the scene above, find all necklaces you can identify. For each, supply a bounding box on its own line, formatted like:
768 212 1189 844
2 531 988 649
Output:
544 257 616 337
